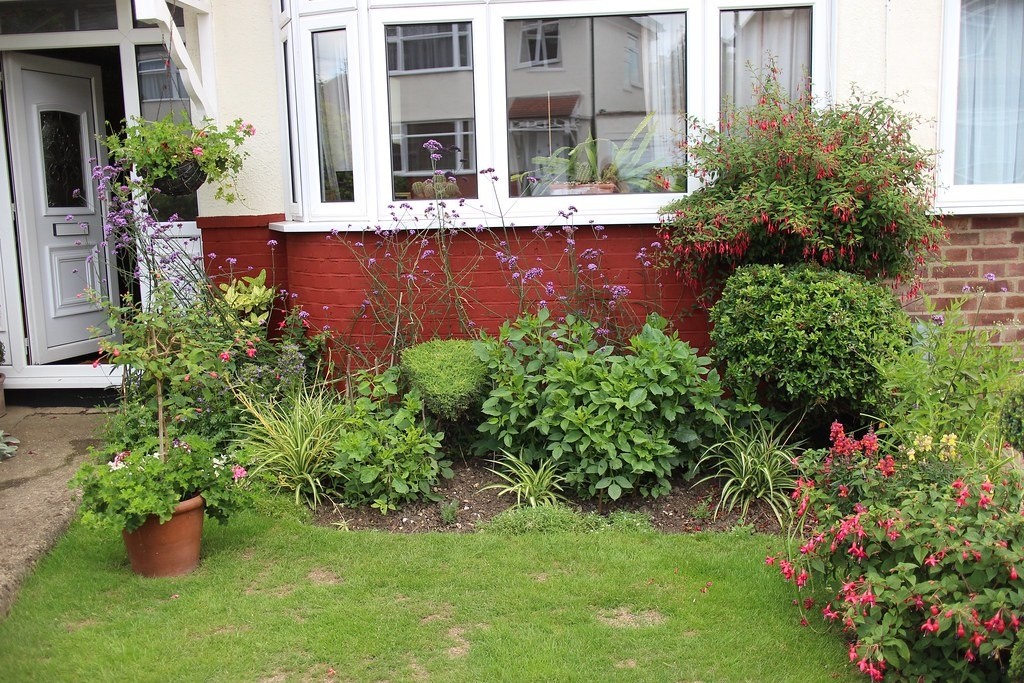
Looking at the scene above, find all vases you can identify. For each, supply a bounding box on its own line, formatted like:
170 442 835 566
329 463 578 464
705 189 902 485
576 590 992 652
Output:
119 493 206 577
140 160 209 197
551 182 615 195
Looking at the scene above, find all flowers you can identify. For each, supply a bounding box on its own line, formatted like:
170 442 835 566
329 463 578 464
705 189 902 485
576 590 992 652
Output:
67 270 255 535
510 109 670 193
92 112 256 204
